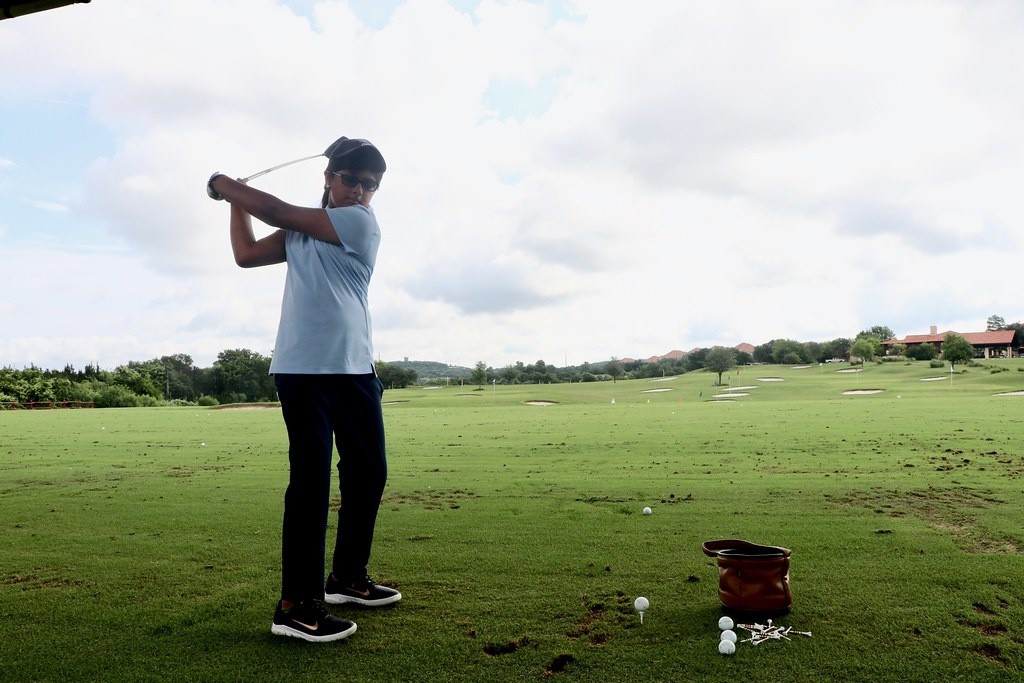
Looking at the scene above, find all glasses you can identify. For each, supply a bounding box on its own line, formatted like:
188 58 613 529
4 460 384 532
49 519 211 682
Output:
331 171 378 191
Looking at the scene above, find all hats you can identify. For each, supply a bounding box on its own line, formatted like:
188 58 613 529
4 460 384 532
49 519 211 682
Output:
327 138 386 179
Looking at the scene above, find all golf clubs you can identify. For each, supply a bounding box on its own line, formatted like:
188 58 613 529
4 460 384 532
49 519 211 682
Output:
243 134 348 182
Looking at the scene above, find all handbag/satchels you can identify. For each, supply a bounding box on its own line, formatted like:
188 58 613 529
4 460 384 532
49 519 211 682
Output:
702 540 792 612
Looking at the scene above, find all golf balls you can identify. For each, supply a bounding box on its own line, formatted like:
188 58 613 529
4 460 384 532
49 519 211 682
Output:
643 507 652 514
718 630 737 654
634 597 650 611
718 616 734 630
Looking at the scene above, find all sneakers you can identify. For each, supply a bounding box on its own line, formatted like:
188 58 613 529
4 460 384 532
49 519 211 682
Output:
324 568 402 606
270 599 358 642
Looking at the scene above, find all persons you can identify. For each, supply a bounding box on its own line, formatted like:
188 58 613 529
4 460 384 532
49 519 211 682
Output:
207 136 402 642
